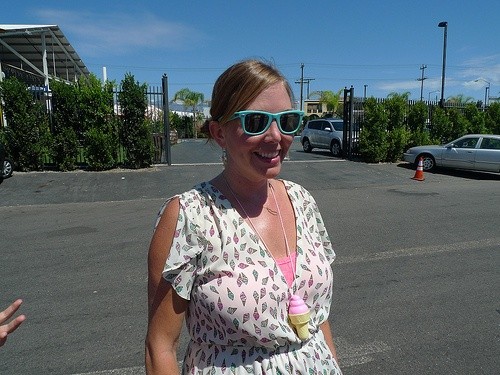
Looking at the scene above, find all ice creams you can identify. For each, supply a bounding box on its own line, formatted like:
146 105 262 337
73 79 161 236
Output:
288 295 311 340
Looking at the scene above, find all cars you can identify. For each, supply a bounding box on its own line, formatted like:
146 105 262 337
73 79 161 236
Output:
404 134 500 172
0 141 13 182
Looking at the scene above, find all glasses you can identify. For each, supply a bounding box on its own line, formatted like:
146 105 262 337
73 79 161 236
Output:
217 110 304 135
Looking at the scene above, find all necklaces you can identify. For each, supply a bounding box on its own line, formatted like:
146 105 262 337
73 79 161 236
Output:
226 178 310 340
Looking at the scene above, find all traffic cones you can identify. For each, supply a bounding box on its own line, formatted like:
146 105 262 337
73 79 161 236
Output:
410 157 425 181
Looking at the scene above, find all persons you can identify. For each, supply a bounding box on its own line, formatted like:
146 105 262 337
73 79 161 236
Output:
0 298 27 346
144 60 348 375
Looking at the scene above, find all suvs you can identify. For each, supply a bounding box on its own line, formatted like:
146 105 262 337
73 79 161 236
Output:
300 118 359 156
